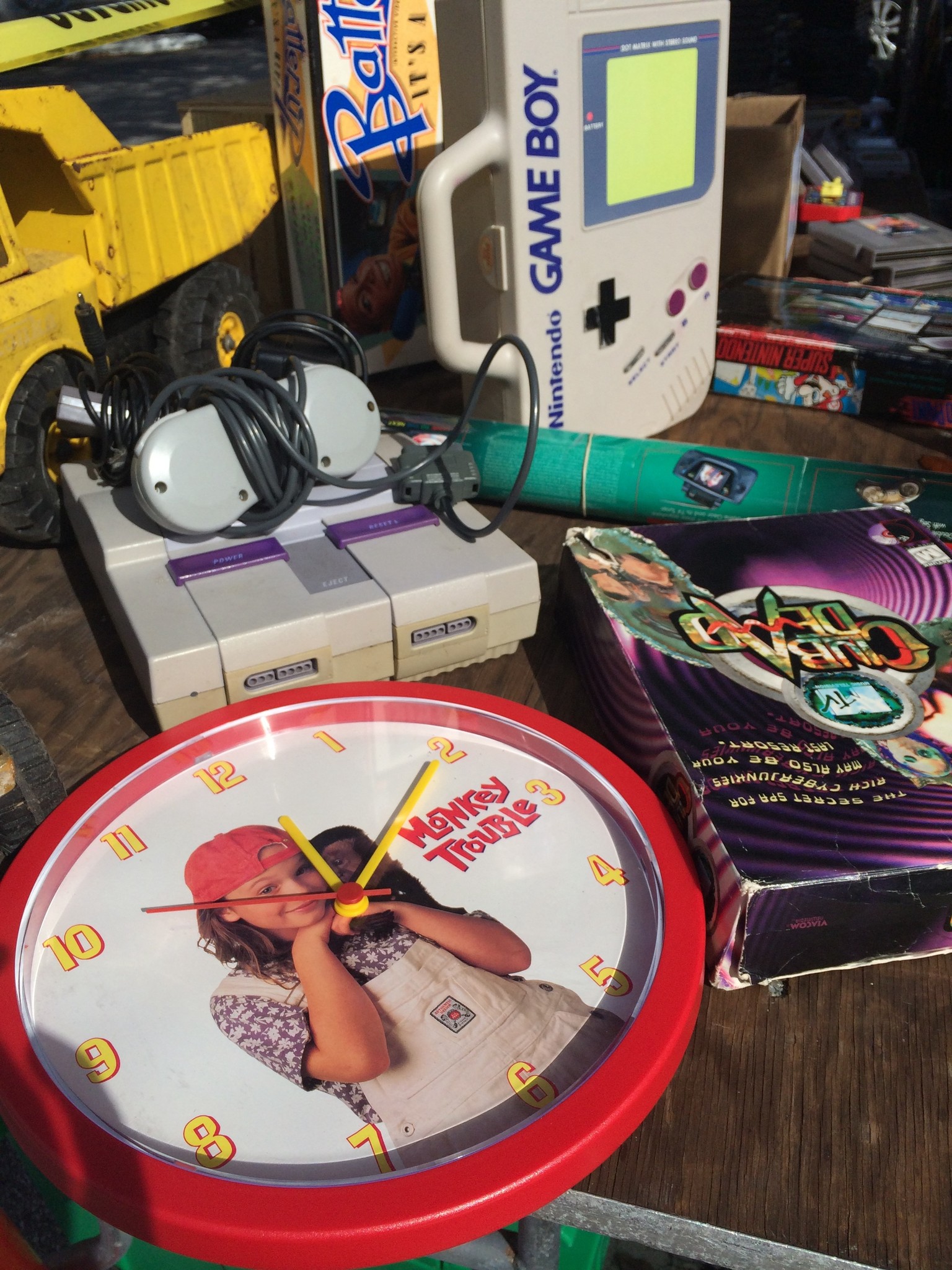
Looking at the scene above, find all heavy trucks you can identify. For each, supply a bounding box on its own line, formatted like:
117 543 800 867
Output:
1 77 284 548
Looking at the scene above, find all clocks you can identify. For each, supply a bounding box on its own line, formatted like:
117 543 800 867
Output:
0 679 706 1270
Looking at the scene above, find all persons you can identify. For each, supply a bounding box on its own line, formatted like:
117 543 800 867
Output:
183 824 626 1170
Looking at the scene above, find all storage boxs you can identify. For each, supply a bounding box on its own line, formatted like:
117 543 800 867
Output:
555 509 945 992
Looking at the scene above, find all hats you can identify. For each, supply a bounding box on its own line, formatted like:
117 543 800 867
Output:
185 824 303 904
